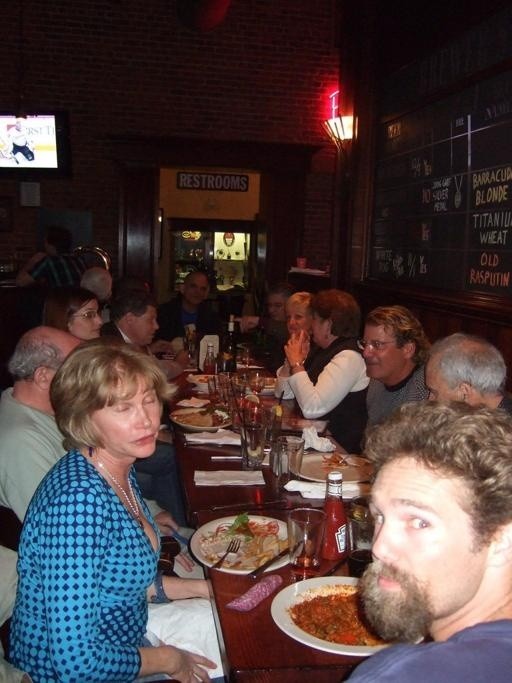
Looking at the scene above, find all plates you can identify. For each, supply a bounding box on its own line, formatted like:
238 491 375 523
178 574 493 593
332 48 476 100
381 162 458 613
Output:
169 407 231 433
290 451 379 483
188 514 304 576
271 576 424 658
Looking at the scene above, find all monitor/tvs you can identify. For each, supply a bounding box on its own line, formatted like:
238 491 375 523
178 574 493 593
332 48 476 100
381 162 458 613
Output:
0 103 71 176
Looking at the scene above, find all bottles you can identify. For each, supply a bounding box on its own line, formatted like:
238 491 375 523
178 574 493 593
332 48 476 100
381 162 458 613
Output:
320 472 349 559
164 313 257 375
0 263 18 273
176 247 240 285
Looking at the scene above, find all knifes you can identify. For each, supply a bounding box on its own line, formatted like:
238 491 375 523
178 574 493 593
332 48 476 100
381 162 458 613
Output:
249 542 303 579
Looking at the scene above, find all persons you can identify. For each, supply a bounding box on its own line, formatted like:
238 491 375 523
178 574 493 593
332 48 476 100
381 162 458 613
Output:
338 397 512 682
7 337 224 682
41 263 369 528
423 331 512 420
1 324 208 656
355 305 432 428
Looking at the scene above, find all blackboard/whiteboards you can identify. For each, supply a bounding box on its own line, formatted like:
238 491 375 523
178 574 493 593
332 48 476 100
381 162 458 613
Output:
360 71 512 328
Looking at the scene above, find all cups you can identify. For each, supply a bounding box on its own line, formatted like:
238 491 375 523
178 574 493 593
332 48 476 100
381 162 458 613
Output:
195 369 304 490
347 494 377 576
297 257 307 269
287 509 329 571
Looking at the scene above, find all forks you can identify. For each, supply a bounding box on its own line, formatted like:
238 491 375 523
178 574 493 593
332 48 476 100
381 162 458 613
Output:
214 537 242 571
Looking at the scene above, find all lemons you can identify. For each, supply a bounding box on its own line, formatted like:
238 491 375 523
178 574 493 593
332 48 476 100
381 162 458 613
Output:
270 405 283 416
244 394 260 404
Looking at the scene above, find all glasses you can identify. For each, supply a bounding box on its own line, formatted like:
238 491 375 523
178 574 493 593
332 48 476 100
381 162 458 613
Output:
70 309 102 321
356 337 405 353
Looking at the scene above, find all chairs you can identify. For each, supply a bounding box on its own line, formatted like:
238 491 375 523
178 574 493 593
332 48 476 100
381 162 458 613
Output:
0 505 182 683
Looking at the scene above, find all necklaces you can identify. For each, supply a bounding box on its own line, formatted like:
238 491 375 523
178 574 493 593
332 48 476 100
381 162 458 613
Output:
100 458 145 530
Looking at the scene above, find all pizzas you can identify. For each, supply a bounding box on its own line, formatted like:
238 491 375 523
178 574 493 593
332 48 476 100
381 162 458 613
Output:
321 455 349 470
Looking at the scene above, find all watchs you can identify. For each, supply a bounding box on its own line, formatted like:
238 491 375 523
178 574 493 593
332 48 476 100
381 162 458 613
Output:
145 568 170 602
